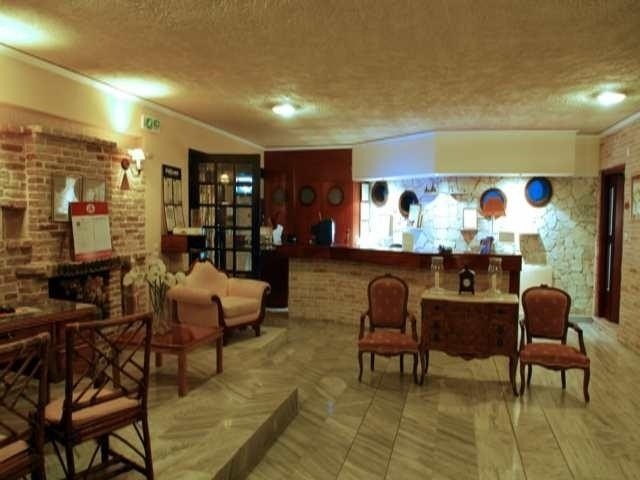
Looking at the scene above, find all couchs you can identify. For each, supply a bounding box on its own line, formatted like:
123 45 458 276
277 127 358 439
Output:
167 258 270 344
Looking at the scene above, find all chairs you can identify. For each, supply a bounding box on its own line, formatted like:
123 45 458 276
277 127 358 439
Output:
357 274 425 384
1 332 51 480
513 285 592 401
30 313 155 478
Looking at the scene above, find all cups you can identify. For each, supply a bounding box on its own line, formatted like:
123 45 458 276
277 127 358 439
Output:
460 229 478 254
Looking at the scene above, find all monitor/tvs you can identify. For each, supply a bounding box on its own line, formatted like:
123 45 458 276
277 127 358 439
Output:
310 217 336 245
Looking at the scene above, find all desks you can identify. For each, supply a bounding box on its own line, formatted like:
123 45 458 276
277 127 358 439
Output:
111 316 223 398
1 300 100 382
284 242 522 327
418 284 521 395
